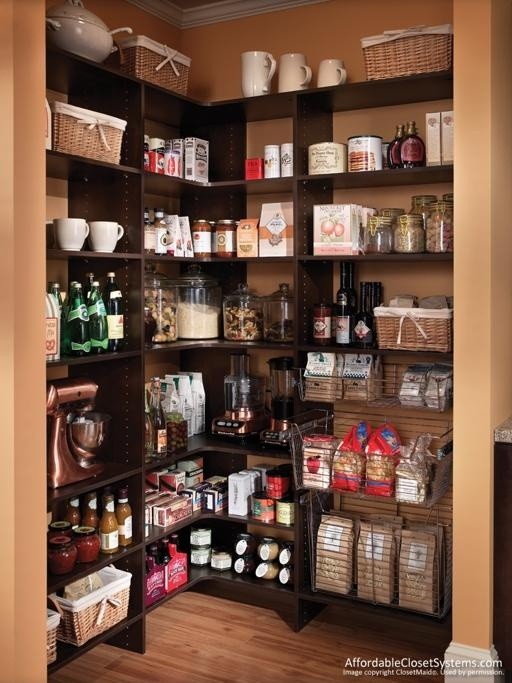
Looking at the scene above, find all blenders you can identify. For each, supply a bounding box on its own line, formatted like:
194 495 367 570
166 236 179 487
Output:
211 351 335 452
46 380 112 491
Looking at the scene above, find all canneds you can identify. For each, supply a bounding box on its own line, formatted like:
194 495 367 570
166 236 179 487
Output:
191 217 240 259
47 519 100 575
190 526 295 587
250 464 295 525
363 193 453 253
311 303 337 347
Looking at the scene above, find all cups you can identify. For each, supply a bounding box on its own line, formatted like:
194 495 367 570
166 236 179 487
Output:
316 58 346 87
53 216 91 254
276 54 311 90
242 49 277 96
84 219 125 254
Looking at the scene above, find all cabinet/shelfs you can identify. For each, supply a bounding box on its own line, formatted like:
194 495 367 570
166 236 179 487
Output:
47 41 452 673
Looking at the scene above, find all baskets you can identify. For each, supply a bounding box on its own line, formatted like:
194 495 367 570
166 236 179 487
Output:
291 362 452 413
282 410 453 507
359 24 454 80
51 102 127 165
47 565 132 667
373 307 452 353
310 501 451 617
103 36 192 97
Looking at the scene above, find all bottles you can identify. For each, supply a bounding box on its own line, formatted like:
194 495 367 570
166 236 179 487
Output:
66 483 135 555
150 207 167 256
142 261 298 344
143 533 189 576
311 259 382 348
143 376 170 465
400 120 425 165
365 190 452 255
144 207 159 256
387 123 405 168
46 270 129 363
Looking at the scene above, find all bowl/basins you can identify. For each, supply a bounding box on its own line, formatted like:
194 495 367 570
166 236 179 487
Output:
44 0 119 63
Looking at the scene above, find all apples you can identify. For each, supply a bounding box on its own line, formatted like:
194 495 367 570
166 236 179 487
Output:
307 456 320 473
